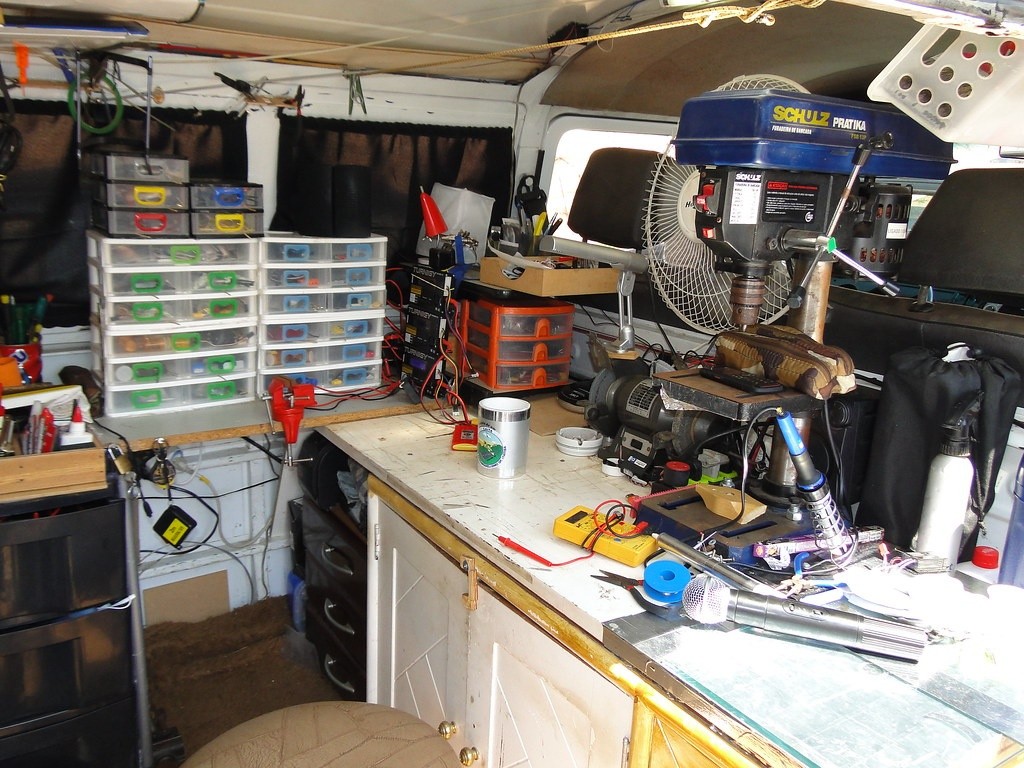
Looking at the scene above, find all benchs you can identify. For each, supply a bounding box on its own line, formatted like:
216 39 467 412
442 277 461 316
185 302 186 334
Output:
566 148 1023 382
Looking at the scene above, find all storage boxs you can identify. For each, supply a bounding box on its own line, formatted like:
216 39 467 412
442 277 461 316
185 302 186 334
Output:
91 213 187 237
191 211 265 237
454 302 577 388
74 146 189 183
190 182 264 210
84 236 387 418
83 180 188 210
479 253 617 295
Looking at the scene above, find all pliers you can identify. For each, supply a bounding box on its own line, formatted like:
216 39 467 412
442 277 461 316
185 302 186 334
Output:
590 568 671 616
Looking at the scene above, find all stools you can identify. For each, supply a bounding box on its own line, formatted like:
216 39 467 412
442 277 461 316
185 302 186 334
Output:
177 702 461 768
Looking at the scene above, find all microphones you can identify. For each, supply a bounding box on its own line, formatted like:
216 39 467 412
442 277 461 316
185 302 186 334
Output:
682 574 928 663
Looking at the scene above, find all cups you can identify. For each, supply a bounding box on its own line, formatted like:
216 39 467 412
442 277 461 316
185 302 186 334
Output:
477 397 531 478
1 343 43 388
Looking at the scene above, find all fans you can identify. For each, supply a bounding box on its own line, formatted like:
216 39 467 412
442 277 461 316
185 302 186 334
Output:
640 74 810 334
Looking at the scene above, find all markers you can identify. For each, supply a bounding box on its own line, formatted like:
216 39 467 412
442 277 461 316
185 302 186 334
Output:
20 414 45 454
0 295 49 345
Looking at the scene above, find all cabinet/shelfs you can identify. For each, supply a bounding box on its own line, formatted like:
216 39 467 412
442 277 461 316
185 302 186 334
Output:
361 478 756 768
0 494 145 768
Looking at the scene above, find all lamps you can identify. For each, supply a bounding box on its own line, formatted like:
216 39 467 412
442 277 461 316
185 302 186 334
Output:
0 14 150 53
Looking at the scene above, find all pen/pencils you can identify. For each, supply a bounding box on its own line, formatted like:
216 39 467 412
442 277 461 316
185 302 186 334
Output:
651 531 786 599
523 213 563 255
